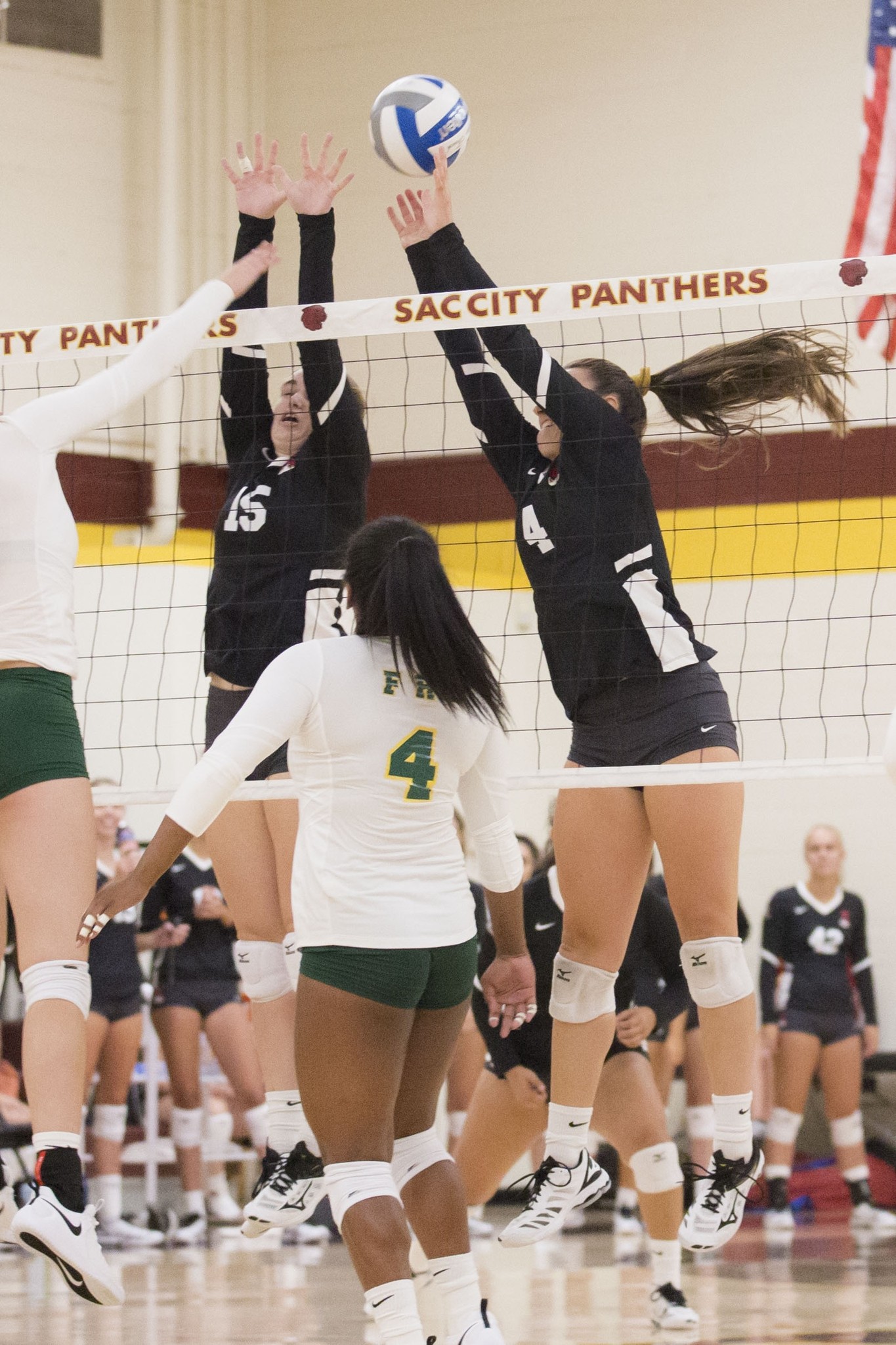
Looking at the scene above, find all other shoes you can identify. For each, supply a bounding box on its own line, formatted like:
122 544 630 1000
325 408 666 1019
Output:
847 1203 896 1227
94 1212 165 1246
171 1212 208 1246
613 1204 642 1237
205 1195 247 1223
761 1206 793 1228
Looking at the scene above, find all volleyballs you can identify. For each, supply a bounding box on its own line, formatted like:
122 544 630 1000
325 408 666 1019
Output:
368 74 470 177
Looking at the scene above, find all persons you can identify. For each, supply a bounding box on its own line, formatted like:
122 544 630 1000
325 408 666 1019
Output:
388 147 858 1258
74 517 539 1345
0 241 282 1299
2 779 896 1247
201 136 373 1240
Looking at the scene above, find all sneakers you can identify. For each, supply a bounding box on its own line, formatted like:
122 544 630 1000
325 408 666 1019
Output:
240 1133 326 1239
11 1175 127 1308
678 1134 766 1252
496 1145 611 1247
648 1281 698 1331
